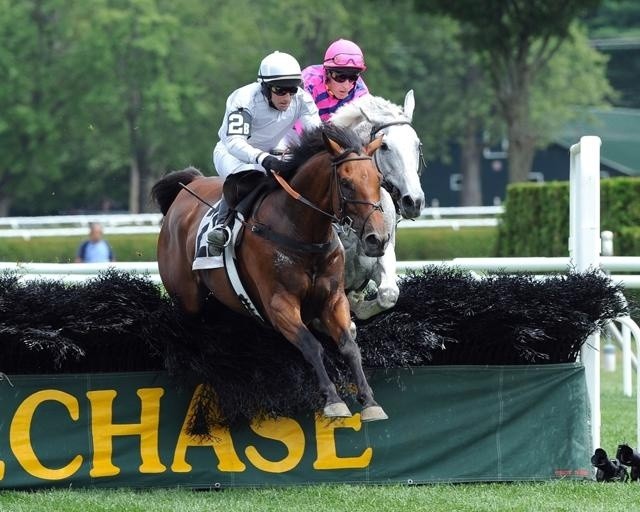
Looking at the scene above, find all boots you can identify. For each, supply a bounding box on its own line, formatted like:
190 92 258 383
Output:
207 200 231 245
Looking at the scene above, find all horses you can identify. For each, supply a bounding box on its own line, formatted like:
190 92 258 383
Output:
326 86 430 312
148 119 391 424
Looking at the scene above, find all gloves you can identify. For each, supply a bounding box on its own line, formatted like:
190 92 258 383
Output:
261 155 290 179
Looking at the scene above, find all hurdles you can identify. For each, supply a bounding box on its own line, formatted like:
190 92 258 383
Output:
0 134 601 493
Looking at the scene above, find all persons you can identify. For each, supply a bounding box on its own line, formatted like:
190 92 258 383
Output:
73 221 117 263
205 50 328 257
272 39 371 162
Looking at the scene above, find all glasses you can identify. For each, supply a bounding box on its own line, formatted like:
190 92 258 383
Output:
324 53 363 65
331 73 358 82
269 86 298 96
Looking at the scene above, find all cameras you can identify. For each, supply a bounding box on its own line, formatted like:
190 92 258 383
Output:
618 445 640 480
591 448 626 482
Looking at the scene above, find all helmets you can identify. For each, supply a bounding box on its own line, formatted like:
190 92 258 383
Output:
324 38 367 72
257 50 302 83
